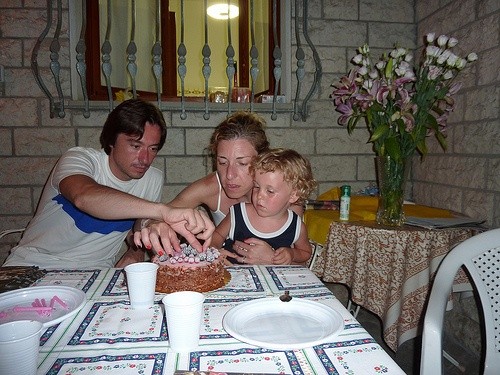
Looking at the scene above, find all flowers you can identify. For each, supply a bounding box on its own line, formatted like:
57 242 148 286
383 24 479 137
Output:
330 30 480 217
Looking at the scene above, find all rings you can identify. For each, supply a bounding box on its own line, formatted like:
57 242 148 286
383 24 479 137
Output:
242 258 245 264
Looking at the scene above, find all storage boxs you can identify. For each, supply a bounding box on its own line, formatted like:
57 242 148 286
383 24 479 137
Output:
256 95 286 103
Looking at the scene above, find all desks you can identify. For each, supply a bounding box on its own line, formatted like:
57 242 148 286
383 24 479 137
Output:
304 185 487 375
0 265 403 375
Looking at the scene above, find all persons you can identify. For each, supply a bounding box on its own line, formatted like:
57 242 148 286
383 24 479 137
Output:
2 99 216 270
211 147 317 266
134 113 306 266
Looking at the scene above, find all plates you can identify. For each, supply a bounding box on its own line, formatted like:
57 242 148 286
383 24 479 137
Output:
221 296 345 351
0 286 87 328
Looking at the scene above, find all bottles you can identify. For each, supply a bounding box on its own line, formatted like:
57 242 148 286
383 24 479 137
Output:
339 185 352 224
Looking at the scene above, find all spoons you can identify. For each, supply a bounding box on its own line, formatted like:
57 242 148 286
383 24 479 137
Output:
279 291 292 302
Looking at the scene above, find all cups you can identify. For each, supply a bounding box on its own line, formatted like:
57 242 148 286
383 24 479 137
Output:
0 320 44 375
162 290 206 352
124 262 160 309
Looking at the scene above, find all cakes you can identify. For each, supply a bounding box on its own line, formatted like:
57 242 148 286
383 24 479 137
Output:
149 243 224 291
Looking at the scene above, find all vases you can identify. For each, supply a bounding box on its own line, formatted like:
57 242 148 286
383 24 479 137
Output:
375 157 415 225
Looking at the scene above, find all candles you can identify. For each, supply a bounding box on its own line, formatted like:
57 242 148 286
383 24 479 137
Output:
0 296 70 319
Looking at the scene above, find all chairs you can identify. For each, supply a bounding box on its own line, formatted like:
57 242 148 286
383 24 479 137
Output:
421 228 500 375
